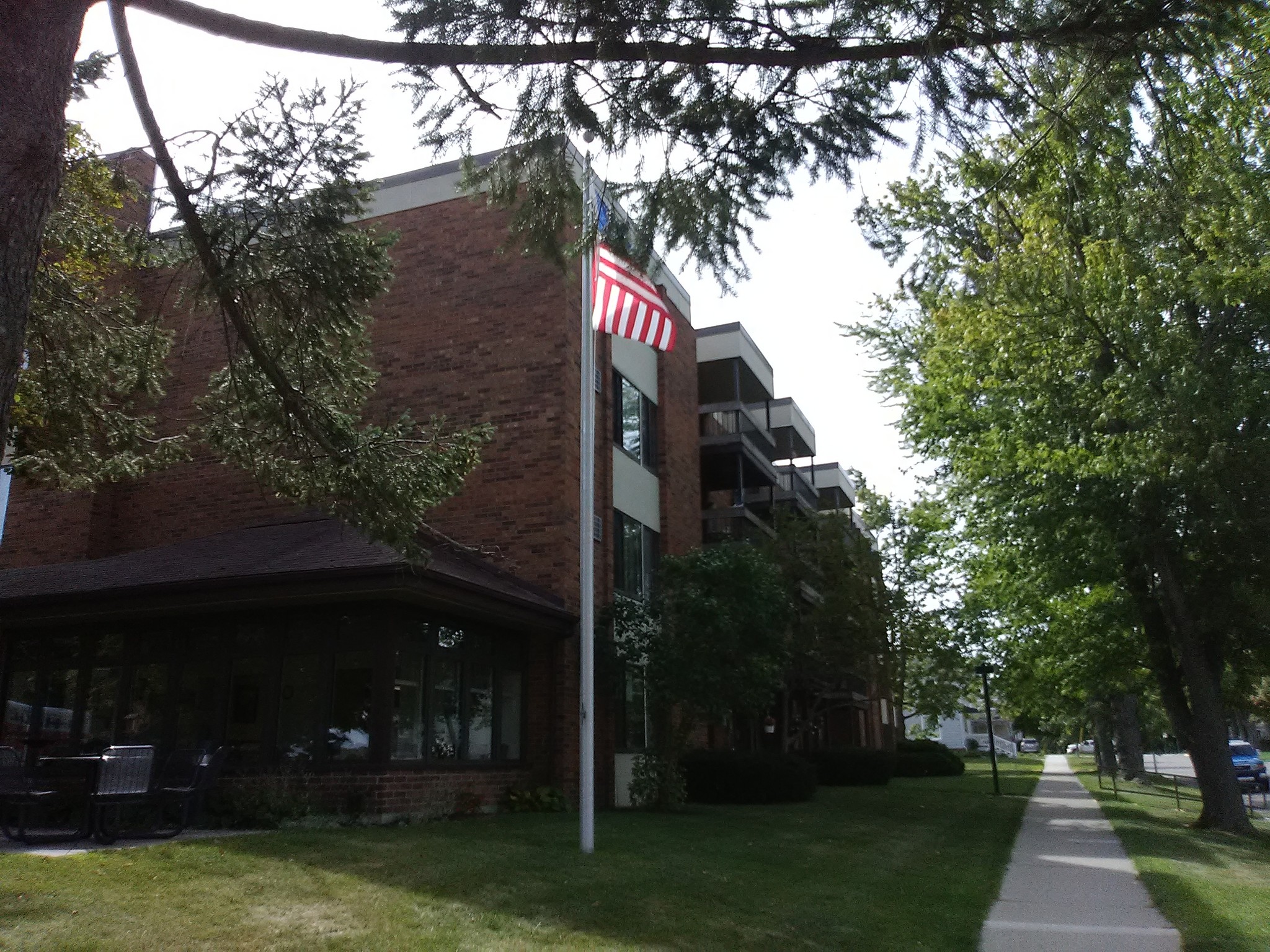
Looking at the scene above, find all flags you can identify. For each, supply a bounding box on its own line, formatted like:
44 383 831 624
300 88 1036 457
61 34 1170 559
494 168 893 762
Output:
592 191 677 351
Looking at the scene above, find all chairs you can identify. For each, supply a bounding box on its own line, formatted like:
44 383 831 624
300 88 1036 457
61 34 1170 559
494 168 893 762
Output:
0 746 237 844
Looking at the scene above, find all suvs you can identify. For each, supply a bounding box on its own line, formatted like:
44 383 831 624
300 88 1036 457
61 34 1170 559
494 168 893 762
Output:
1020 738 1039 753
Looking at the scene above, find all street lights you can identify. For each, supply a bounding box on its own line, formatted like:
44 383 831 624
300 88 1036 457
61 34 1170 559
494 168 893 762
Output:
973 660 1000 796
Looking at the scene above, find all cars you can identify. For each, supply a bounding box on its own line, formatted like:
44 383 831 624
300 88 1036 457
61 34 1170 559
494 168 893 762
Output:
1067 739 1095 755
1228 739 1266 777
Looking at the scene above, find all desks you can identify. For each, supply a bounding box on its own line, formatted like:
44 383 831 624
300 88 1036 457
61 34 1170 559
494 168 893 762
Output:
37 755 152 836
